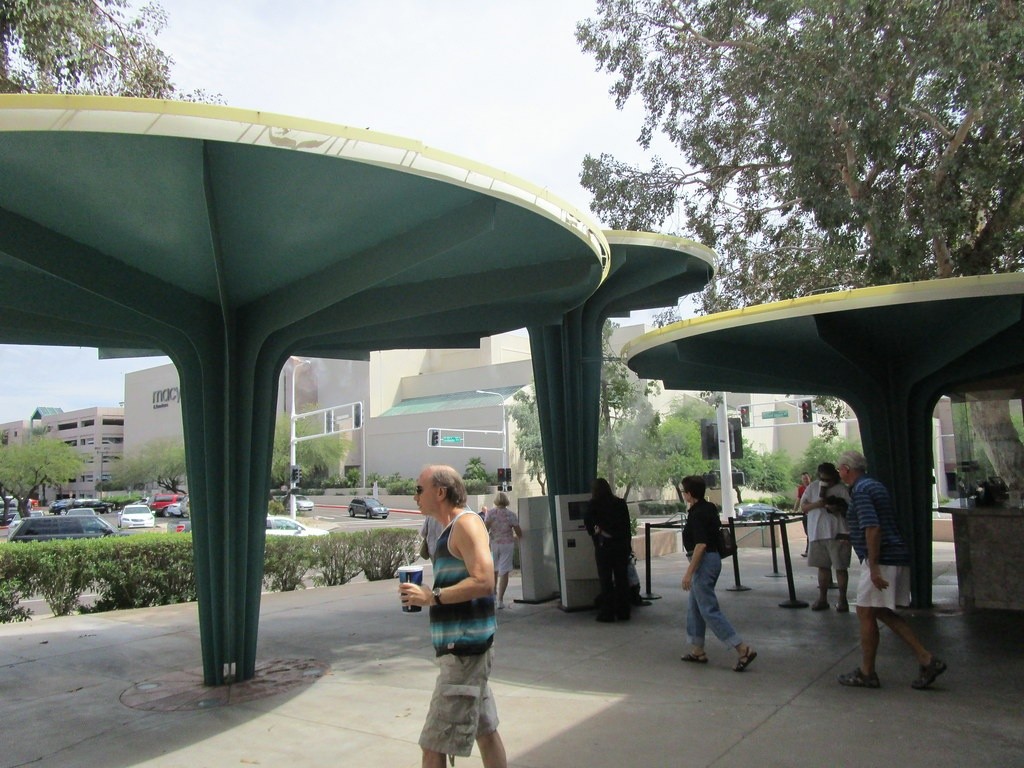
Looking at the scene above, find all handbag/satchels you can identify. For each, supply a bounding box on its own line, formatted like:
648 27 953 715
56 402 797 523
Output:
717 516 738 559
594 589 632 623
419 518 430 560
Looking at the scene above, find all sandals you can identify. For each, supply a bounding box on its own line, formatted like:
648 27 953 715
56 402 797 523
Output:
911 655 947 689
732 647 757 671
680 652 709 664
837 667 882 688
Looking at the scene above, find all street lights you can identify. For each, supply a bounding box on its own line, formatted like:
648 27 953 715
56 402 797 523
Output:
476 390 507 494
288 359 312 519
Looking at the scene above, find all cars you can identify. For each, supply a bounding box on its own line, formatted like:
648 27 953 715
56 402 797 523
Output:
265 516 329 537
346 496 390 519
167 517 192 533
118 504 156 529
733 502 789 522
65 507 100 516
283 495 314 511
0 491 190 526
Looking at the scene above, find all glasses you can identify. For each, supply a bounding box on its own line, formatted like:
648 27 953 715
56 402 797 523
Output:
680 489 686 493
817 475 835 483
416 485 448 496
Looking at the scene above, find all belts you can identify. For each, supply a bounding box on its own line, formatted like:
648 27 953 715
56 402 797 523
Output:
686 548 716 557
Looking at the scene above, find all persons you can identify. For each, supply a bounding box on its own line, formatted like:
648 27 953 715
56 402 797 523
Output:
398 465 507 768
478 506 487 520
680 476 757 672
484 492 522 609
584 477 641 622
420 515 443 563
837 450 947 690
800 463 853 611
792 473 811 557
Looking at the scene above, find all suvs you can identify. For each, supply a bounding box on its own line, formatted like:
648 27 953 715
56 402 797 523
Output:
7 514 143 543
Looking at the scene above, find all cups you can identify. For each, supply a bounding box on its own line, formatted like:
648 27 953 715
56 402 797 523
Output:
397 565 424 614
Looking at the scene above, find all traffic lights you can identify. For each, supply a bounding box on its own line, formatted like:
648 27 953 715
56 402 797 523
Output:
498 468 504 482
508 486 512 491
506 468 511 481
801 400 812 422
498 486 502 491
741 406 750 427
290 464 303 483
432 429 439 446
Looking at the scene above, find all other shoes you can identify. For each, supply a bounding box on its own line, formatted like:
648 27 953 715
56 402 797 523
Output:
812 601 831 611
832 603 849 612
496 601 505 608
800 552 809 557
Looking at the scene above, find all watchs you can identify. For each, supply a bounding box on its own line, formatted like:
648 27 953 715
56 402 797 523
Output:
432 588 442 604
